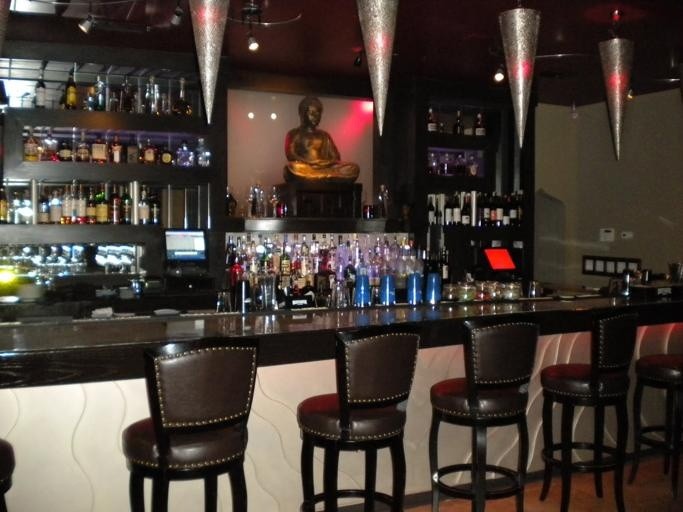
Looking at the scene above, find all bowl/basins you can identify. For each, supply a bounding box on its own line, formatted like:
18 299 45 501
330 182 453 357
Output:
13 285 40 303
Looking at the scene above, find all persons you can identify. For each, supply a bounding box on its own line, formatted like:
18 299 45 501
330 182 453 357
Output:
278 93 361 183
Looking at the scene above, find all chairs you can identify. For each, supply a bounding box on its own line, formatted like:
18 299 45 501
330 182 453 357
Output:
427 313 537 512
118 341 257 511
298 314 424 512
537 308 638 512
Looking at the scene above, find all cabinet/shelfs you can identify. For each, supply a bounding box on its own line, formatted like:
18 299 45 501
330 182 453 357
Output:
0 41 227 303
371 95 534 281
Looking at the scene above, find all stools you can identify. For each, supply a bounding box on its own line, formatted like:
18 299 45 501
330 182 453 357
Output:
627 352 681 500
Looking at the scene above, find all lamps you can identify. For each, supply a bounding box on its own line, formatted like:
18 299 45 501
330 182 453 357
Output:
75 14 97 34
238 0 263 51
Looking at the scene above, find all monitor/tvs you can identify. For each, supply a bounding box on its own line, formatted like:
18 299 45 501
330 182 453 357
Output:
164 228 207 261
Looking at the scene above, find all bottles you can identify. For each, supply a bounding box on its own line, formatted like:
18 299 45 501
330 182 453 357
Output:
445 303 539 318
664 272 672 284
225 185 287 218
219 304 445 336
427 152 479 177
473 110 485 137
224 236 448 314
620 267 653 296
1 73 210 276
453 110 463 135
424 191 522 229
443 282 542 303
426 107 439 133
361 185 394 220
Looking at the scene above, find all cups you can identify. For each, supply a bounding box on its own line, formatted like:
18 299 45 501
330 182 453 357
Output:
669 264 682 283
